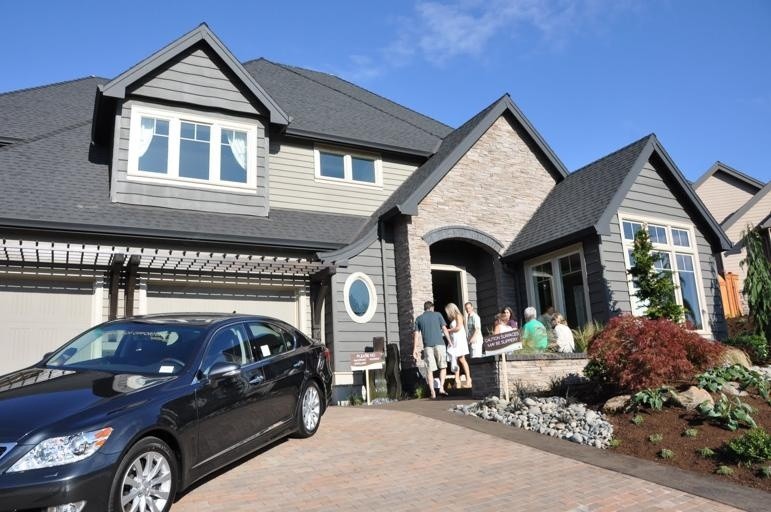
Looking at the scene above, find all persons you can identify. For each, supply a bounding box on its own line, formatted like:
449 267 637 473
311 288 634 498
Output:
414 301 575 400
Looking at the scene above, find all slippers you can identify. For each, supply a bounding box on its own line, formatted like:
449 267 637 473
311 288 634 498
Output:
428 379 473 400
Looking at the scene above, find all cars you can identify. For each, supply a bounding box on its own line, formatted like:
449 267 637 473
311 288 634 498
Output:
0 310 336 512
